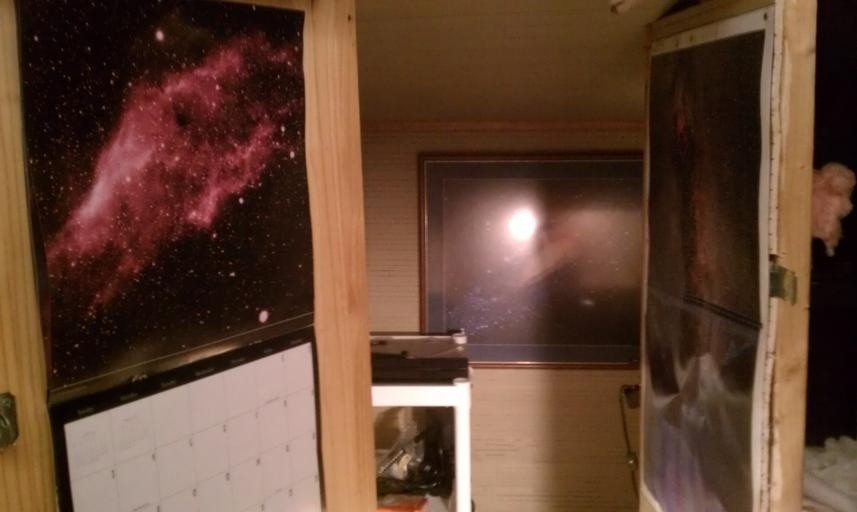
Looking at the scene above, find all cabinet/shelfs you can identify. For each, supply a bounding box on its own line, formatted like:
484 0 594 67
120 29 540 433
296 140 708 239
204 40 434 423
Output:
370 329 473 511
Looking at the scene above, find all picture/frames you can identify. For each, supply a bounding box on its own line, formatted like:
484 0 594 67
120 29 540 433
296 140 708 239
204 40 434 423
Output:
416 146 645 371
